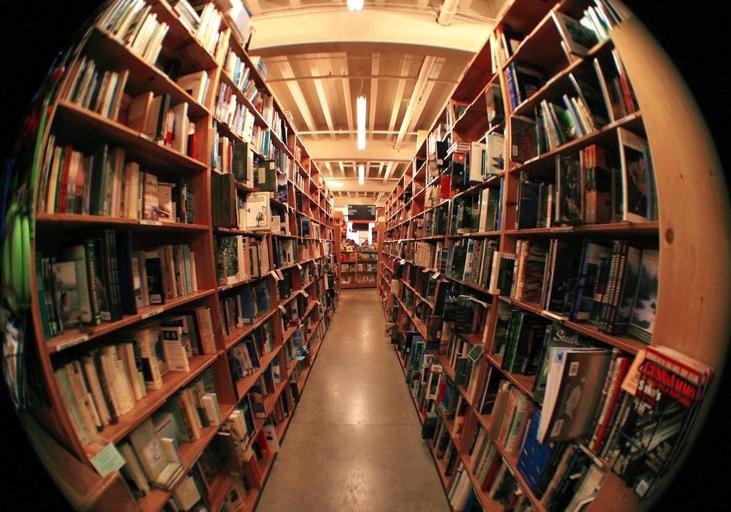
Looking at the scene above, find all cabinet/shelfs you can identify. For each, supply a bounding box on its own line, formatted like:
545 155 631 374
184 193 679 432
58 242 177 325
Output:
334 216 346 246
0 0 342 511
376 0 730 511
367 227 377 247
340 251 377 289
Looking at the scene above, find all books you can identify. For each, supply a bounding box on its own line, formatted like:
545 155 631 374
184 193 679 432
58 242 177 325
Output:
380 34 506 511
493 1 714 511
37 0 253 510
342 243 377 285
213 46 337 451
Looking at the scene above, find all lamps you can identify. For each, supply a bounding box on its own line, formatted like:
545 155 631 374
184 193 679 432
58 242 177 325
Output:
435 0 459 28
357 95 366 150
